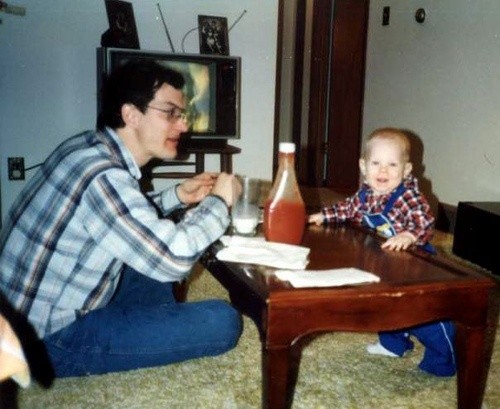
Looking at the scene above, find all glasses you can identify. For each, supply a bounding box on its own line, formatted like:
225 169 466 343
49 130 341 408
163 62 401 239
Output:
146 100 197 126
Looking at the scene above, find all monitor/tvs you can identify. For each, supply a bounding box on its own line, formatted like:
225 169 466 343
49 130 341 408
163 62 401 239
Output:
95 47 243 147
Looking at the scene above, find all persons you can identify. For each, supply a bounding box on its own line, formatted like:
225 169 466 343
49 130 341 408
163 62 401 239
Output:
202 21 225 54
305 128 458 380
0 57 243 377
114 8 133 46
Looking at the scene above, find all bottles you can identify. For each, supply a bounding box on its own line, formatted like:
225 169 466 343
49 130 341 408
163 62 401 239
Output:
263 141 306 245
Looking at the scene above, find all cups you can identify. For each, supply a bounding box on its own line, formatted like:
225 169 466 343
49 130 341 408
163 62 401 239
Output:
232 175 261 237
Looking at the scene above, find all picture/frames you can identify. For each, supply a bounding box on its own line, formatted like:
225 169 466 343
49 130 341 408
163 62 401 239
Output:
198 14 231 57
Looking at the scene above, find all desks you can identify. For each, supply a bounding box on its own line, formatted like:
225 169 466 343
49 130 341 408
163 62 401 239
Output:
140 145 240 192
168 204 500 409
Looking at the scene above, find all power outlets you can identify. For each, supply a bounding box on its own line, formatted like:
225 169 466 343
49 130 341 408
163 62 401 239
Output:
7 156 26 180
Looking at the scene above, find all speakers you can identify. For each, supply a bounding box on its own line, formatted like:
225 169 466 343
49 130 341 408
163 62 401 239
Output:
453 200 500 277
